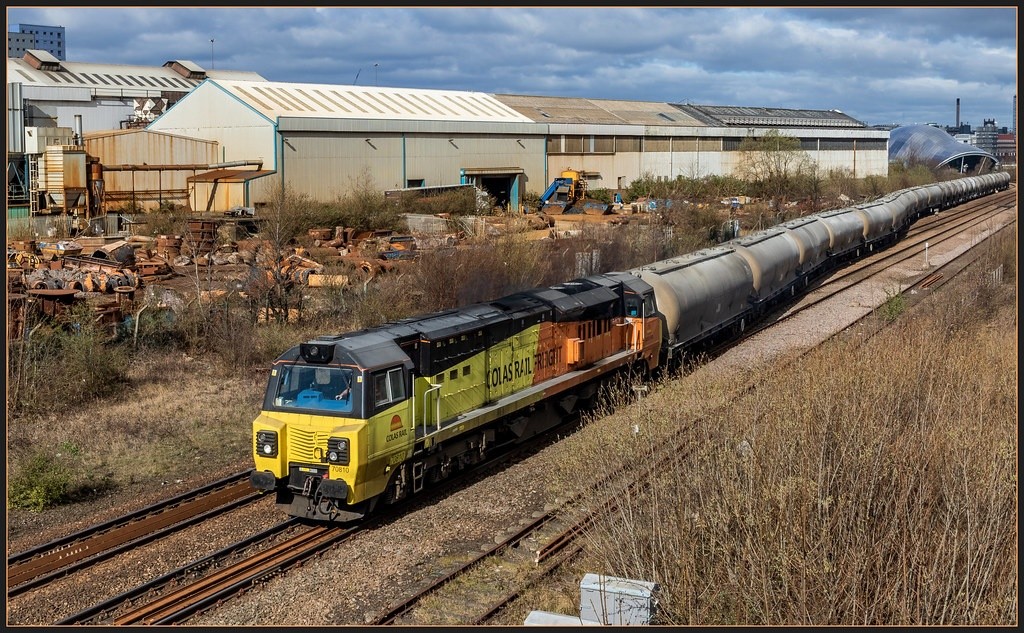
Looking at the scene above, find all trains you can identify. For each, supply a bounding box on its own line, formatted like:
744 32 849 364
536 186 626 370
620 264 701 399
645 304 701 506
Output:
249 170 1011 524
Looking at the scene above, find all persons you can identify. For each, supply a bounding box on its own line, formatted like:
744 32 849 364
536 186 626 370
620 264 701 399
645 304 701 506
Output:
335 387 352 400
